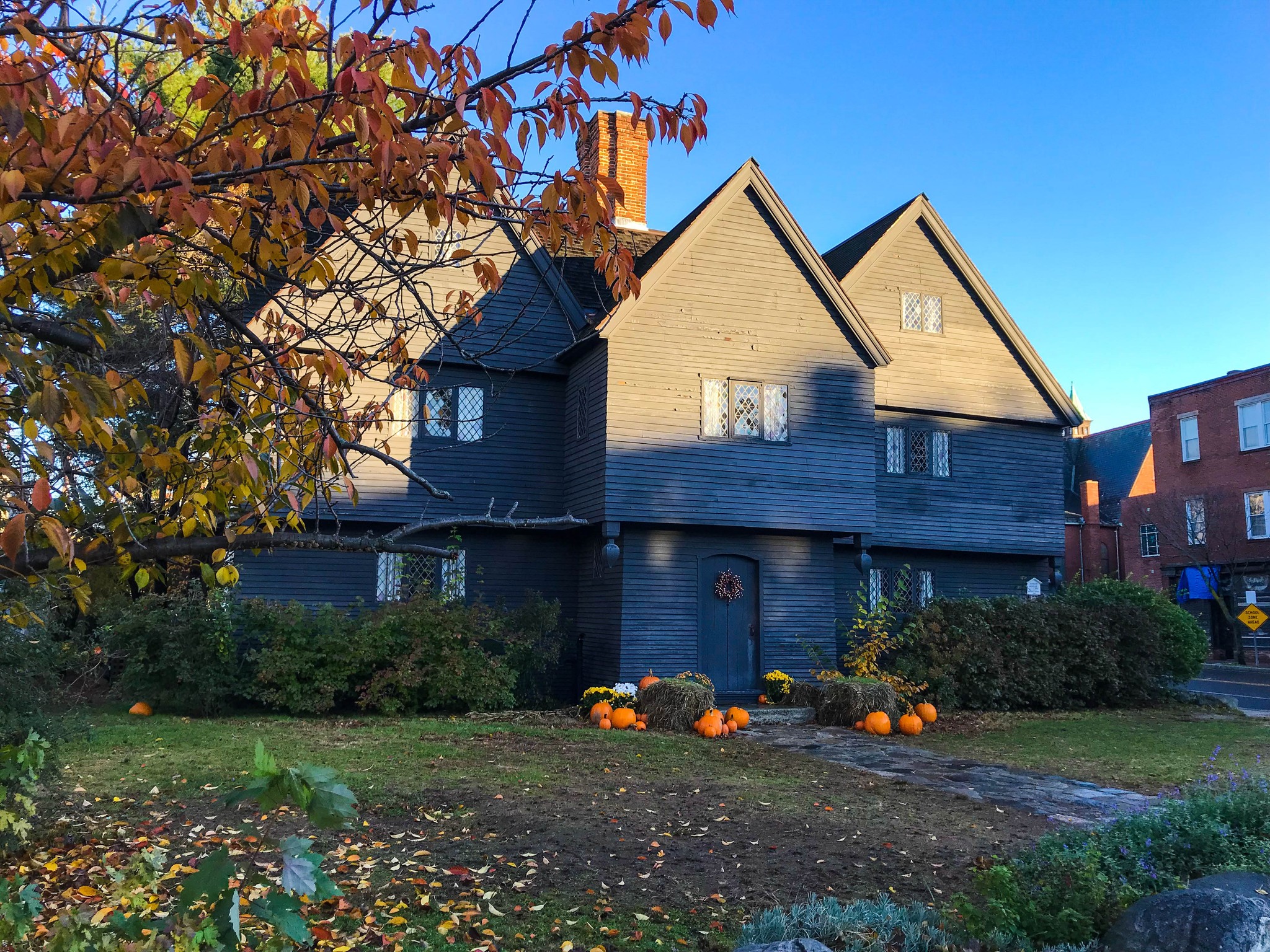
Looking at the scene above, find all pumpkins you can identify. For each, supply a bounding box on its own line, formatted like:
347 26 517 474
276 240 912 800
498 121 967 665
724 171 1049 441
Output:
720 718 729 735
726 714 737 733
638 668 660 689
705 707 724 722
914 699 937 723
599 714 611 729
855 721 865 731
636 713 648 725
693 720 699 729
725 707 750 729
590 700 613 725
129 701 152 715
899 709 923 736
612 702 636 729
865 708 891 735
698 708 722 735
704 725 716 737
630 721 646 731
